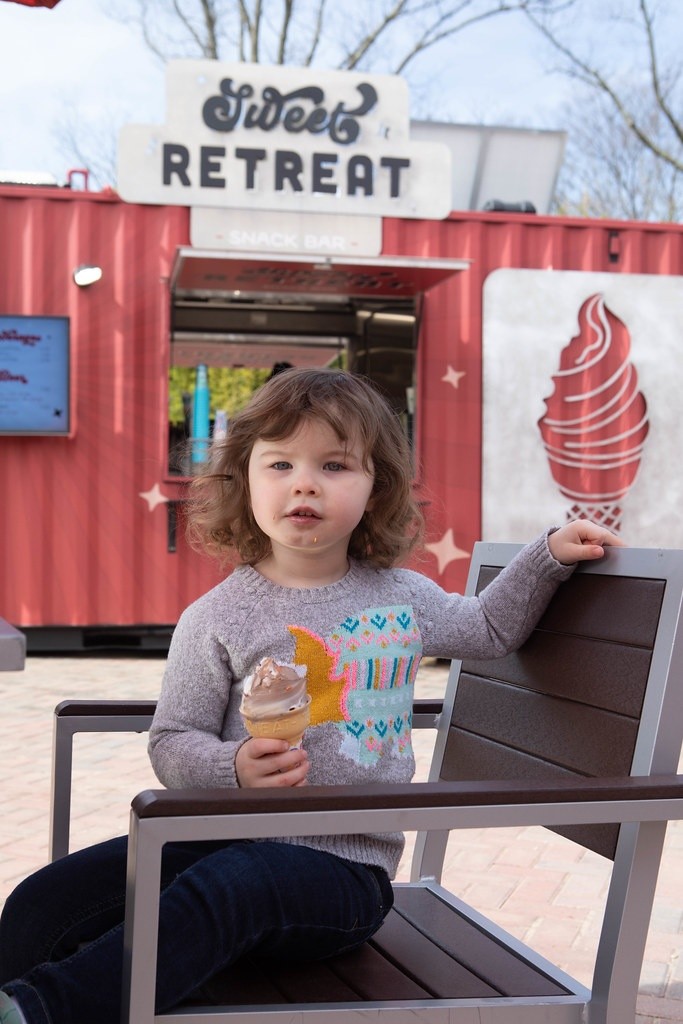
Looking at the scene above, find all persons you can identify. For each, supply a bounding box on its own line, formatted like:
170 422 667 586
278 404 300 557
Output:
0 368 627 1023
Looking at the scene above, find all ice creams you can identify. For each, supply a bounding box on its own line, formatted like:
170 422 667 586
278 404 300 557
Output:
536 293 649 538
240 655 312 774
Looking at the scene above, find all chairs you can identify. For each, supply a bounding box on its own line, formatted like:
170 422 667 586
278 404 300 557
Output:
49 539 682 1024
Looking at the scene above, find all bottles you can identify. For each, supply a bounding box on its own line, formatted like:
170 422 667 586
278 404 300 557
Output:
190 364 212 463
214 408 228 460
